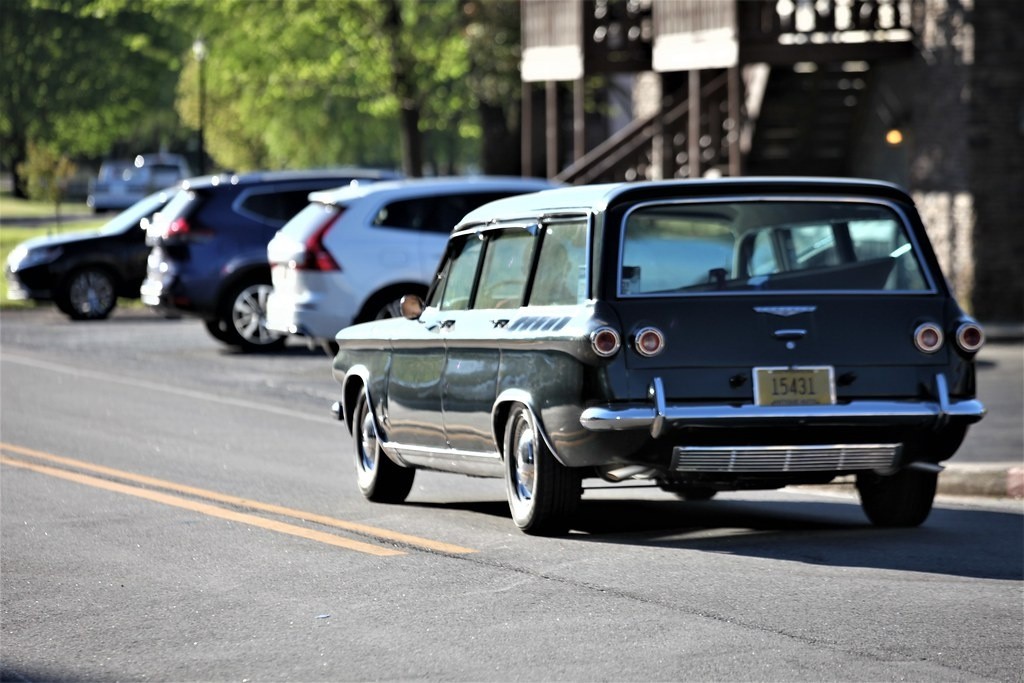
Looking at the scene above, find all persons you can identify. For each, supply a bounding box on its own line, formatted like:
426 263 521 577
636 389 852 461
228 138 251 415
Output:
496 237 577 309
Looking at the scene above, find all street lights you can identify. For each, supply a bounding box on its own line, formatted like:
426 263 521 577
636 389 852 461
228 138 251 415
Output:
193 41 206 174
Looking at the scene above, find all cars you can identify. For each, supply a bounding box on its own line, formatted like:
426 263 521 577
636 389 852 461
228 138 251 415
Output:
89 156 189 215
265 177 773 358
330 177 985 537
6 186 182 322
140 169 405 356
